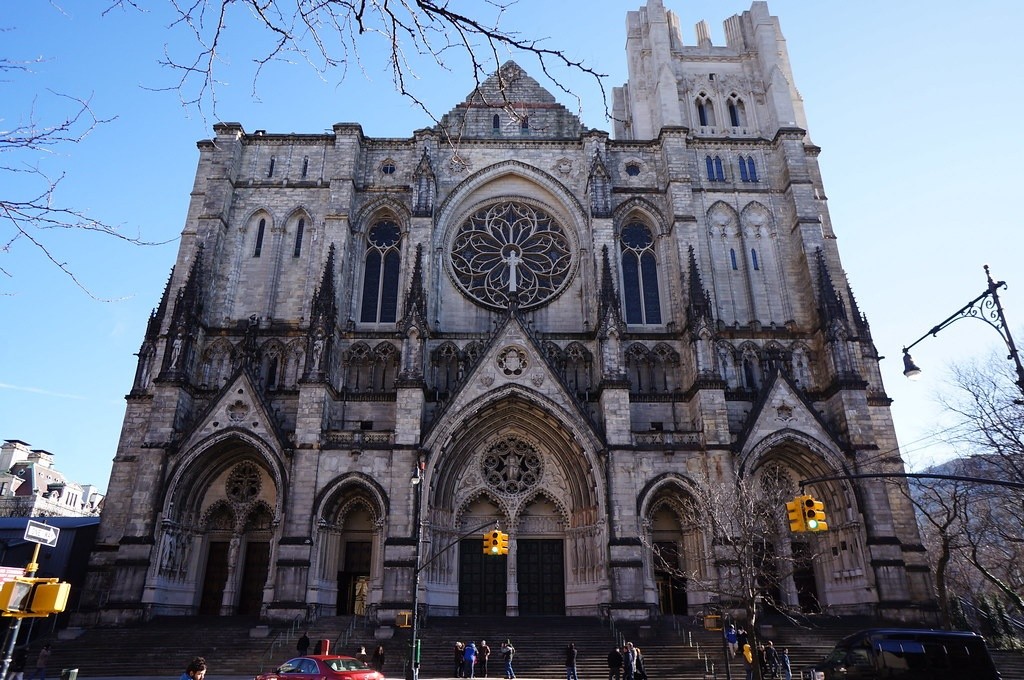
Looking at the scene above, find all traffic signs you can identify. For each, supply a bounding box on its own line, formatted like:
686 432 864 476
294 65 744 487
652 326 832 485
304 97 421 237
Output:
24 520 60 547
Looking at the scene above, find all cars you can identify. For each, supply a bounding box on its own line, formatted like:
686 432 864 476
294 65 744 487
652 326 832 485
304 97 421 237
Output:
254 654 385 680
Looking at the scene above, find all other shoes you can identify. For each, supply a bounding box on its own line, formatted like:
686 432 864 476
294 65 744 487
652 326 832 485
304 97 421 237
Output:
504 675 511 679
771 675 774 680
511 675 516 679
776 673 779 678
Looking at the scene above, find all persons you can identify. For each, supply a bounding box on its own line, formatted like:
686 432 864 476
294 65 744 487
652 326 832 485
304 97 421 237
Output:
566 643 577 680
28 643 52 680
454 640 490 679
765 640 780 679
352 646 368 663
725 624 747 659
501 639 516 679
607 642 647 680
758 645 768 680
8 646 30 680
314 637 322 655
743 644 753 680
297 631 310 656
372 646 385 671
179 656 206 680
503 448 520 481
782 648 792 680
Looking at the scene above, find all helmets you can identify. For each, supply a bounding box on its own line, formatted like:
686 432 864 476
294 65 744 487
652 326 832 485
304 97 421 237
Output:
505 639 510 645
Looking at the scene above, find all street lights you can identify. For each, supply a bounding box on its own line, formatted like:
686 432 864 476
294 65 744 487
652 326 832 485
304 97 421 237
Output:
900 264 1024 396
405 458 424 680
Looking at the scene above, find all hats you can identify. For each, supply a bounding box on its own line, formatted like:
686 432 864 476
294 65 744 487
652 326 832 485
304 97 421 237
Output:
729 624 734 629
481 640 486 644
456 642 461 646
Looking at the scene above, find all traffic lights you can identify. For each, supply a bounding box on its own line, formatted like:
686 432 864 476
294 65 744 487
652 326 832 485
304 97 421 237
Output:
482 530 509 555
703 617 716 629
396 612 407 626
784 497 805 534
0 581 33 614
804 495 828 532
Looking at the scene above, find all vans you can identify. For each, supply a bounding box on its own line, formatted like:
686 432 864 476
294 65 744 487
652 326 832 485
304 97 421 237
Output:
803 628 1002 680
